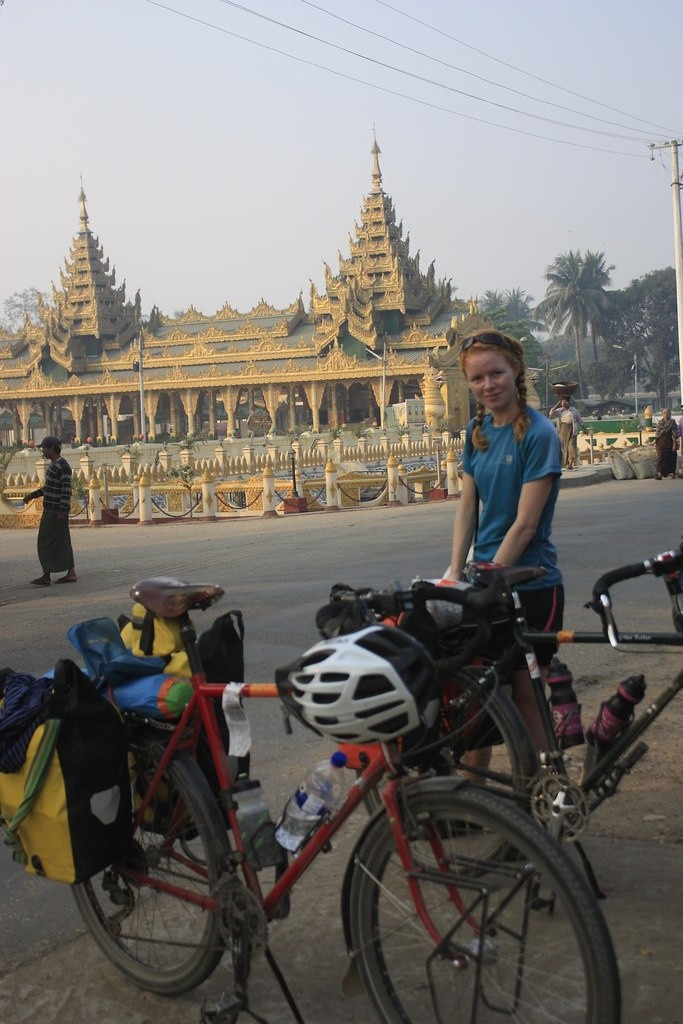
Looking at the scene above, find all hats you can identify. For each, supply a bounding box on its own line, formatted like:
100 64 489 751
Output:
35 436 62 448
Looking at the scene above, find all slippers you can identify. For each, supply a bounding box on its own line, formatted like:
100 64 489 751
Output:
55 578 77 583
30 580 50 585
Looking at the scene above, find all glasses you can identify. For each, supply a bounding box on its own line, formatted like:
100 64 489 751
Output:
458 333 522 358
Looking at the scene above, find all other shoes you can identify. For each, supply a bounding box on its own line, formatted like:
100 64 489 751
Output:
678 474 683 478
566 465 572 470
654 476 662 480
408 820 465 839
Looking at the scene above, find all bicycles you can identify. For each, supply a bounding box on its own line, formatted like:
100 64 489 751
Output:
58 565 627 1024
318 533 683 892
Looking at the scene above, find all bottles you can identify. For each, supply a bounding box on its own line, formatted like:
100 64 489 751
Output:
270 748 348 851
232 770 279 871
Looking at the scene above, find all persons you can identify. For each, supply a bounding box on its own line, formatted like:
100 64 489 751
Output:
548 398 586 469
677 405 683 479
654 409 678 482
20 436 77 585
441 328 564 838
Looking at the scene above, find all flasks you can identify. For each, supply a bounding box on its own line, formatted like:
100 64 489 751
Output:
584 675 644 755
548 656 584 749
